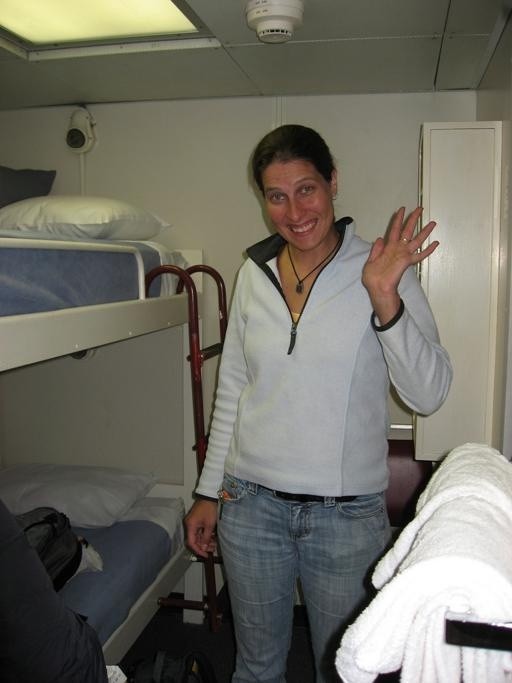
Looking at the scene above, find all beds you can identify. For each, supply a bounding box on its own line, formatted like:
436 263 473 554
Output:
0 237 215 681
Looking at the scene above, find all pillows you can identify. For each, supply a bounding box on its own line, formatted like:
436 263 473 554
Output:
0 192 172 241
0 461 157 528
0 165 55 205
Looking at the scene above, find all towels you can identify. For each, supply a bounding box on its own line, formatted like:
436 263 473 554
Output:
331 443 512 683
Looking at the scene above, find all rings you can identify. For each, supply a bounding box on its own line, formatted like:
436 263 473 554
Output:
400 235 411 243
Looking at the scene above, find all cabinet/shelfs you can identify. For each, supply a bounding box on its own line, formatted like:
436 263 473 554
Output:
412 119 502 460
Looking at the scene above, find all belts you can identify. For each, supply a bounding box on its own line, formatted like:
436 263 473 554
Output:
270 488 360 502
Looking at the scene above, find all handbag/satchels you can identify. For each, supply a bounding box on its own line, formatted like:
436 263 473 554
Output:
126 646 215 682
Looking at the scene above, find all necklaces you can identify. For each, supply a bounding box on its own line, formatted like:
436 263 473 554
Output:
286 233 342 294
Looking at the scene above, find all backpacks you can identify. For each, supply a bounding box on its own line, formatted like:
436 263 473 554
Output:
13 506 88 600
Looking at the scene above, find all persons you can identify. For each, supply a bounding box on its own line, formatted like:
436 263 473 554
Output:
185 122 453 681
